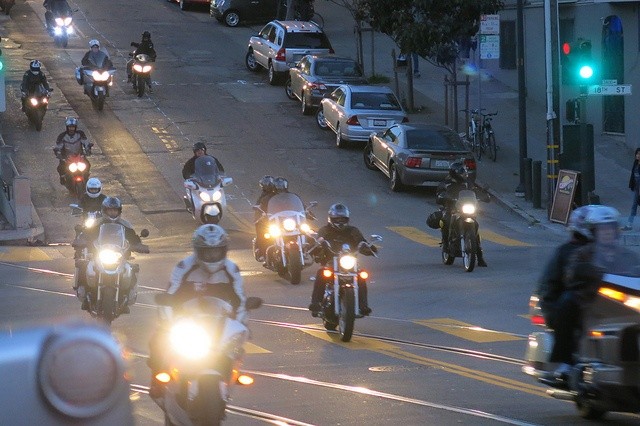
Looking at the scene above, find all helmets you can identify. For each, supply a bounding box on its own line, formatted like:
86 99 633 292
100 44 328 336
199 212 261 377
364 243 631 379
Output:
585 204 621 243
66 116 78 132
102 197 122 222
329 203 349 230
30 60 41 74
427 211 443 231
567 205 594 241
85 178 102 197
449 162 469 184
273 178 288 192
90 40 100 51
194 142 205 154
260 176 275 191
192 225 230 272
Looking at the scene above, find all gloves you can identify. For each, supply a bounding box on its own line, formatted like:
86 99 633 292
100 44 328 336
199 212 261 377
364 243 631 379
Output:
132 242 149 254
72 240 87 252
360 243 378 257
72 209 83 218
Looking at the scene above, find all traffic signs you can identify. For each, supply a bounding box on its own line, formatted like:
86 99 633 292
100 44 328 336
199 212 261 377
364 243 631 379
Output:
481 34 501 59
479 16 501 36
587 85 633 96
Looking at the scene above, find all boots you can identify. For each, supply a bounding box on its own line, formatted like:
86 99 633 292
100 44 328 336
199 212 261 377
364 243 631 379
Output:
477 252 486 267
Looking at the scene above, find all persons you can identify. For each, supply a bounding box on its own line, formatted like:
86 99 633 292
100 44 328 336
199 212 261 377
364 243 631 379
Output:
307 202 374 320
41 0 64 24
146 223 249 398
19 59 53 112
81 38 114 74
533 203 600 388
54 115 92 180
75 195 143 309
74 178 108 217
433 159 492 267
125 31 159 92
258 176 310 265
181 141 225 184
559 205 635 352
251 175 276 203
621 148 639 232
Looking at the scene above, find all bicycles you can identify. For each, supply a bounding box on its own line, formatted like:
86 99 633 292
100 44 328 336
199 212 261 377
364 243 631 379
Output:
461 107 484 162
473 111 501 164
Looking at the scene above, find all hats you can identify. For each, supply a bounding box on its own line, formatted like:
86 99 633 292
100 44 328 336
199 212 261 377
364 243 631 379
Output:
142 31 150 38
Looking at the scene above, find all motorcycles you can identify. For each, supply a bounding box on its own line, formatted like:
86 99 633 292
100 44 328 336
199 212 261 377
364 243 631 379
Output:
44 9 80 49
427 193 492 274
149 285 263 425
124 50 156 98
49 144 96 200
18 89 55 132
67 204 107 229
71 222 148 328
178 157 232 226
250 201 325 285
525 270 640 422
75 65 118 113
299 222 385 342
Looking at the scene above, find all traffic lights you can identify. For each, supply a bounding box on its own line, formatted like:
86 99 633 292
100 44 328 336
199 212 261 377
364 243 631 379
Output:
560 39 600 88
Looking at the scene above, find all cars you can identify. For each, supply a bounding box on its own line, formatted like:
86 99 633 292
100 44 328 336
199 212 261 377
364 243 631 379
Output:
208 1 314 28
317 82 410 149
365 123 475 192
284 55 364 117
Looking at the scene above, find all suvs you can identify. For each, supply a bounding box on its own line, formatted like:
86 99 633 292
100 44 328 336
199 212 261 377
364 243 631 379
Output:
247 13 326 86
